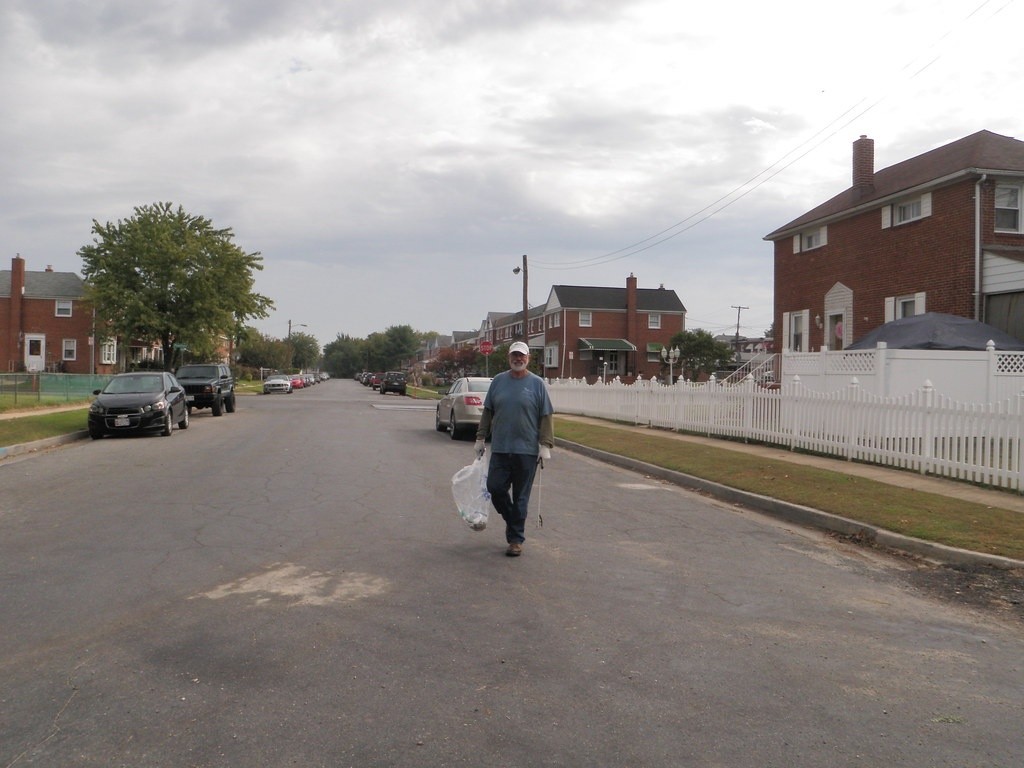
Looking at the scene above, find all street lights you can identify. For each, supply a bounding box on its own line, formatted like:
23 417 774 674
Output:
661 345 680 385
513 267 529 347
288 320 307 348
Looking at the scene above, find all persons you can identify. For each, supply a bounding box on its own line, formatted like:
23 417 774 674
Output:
474 342 554 556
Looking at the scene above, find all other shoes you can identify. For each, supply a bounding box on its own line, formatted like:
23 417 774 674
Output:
506 543 522 555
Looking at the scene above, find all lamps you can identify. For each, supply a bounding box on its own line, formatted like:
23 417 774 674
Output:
814 314 823 330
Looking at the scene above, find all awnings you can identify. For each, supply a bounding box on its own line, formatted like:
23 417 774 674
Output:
578 338 637 351
647 343 663 353
117 335 154 348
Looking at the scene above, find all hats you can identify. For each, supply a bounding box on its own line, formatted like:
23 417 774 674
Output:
508 342 529 354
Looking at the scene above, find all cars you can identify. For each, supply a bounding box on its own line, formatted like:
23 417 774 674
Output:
290 375 304 389
320 373 327 380
264 375 292 394
313 373 321 383
306 374 315 385
88 371 189 439
436 377 495 439
355 372 372 385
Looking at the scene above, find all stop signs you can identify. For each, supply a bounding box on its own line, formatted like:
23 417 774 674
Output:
479 341 494 356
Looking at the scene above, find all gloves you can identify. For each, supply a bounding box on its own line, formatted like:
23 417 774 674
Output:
536 444 551 469
473 438 485 460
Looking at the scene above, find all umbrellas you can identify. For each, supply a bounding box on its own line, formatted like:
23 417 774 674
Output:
845 312 1024 352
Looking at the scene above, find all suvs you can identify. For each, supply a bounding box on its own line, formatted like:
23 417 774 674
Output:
175 363 235 416
372 373 386 390
380 371 407 396
